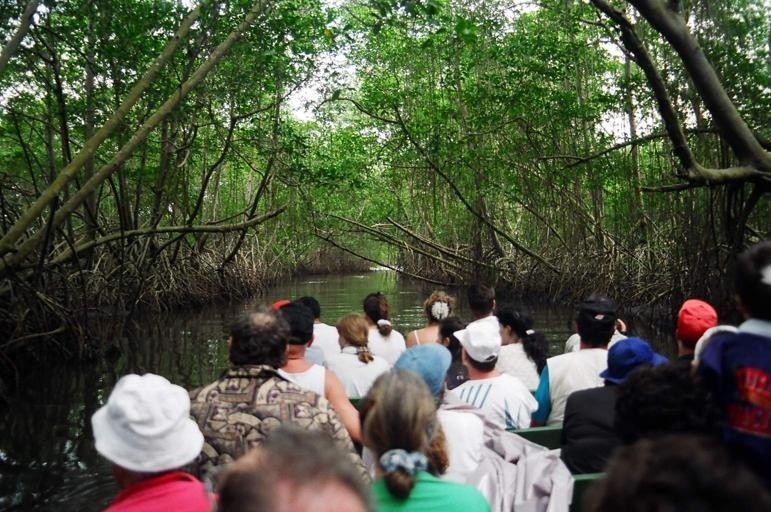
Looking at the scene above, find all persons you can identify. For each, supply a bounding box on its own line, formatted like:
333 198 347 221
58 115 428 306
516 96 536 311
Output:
537 299 618 423
334 309 389 404
362 368 492 511
364 290 406 363
218 432 371 512
293 297 341 361
398 344 484 498
407 290 461 344
501 306 547 391
178 309 367 487
450 319 536 429
435 316 464 353
279 303 362 439
91 372 217 512
453 285 505 362
563 239 771 512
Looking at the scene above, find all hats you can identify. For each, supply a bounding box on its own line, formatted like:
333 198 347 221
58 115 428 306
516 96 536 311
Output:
395 341 453 399
572 296 618 342
89 372 205 475
697 333 771 456
599 336 670 386
272 301 315 346
677 298 720 345
453 315 504 364
